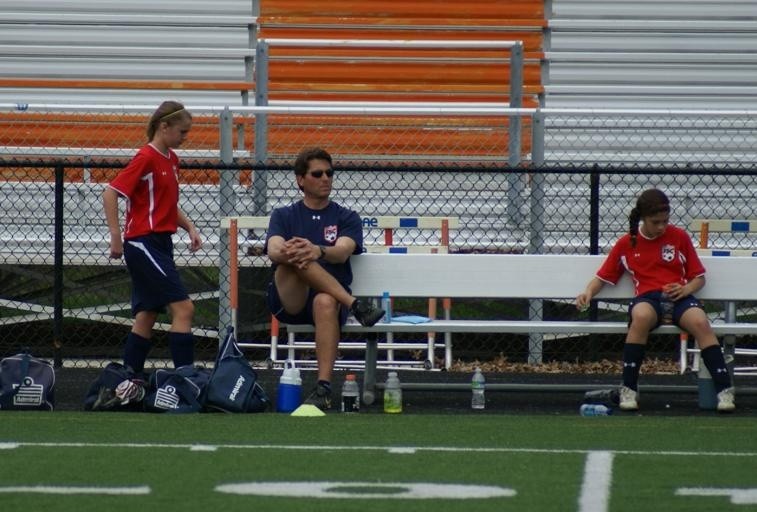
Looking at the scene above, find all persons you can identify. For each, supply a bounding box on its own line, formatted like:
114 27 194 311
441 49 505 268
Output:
100 100 204 384
261 147 386 419
575 188 737 415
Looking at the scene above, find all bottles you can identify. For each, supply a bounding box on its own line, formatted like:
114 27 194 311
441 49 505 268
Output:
341 375 361 413
471 367 486 409
660 287 674 322
383 371 403 413
579 404 613 418
585 389 620 403
381 291 391 324
275 357 303 414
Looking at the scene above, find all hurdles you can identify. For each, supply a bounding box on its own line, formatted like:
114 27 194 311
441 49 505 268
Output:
679 220 756 375
219 216 459 374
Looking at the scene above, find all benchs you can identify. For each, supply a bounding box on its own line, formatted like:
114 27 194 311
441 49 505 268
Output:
284 252 757 416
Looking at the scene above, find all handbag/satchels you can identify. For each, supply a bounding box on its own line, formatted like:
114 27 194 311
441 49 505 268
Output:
1 354 56 411
84 332 272 414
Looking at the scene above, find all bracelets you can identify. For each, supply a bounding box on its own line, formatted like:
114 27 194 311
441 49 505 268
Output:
318 244 327 261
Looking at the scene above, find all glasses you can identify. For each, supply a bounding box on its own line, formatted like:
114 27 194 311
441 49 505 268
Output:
307 168 334 178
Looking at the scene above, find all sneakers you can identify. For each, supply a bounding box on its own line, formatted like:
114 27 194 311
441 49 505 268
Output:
617 382 639 410
304 381 333 410
350 299 385 326
716 386 736 411
